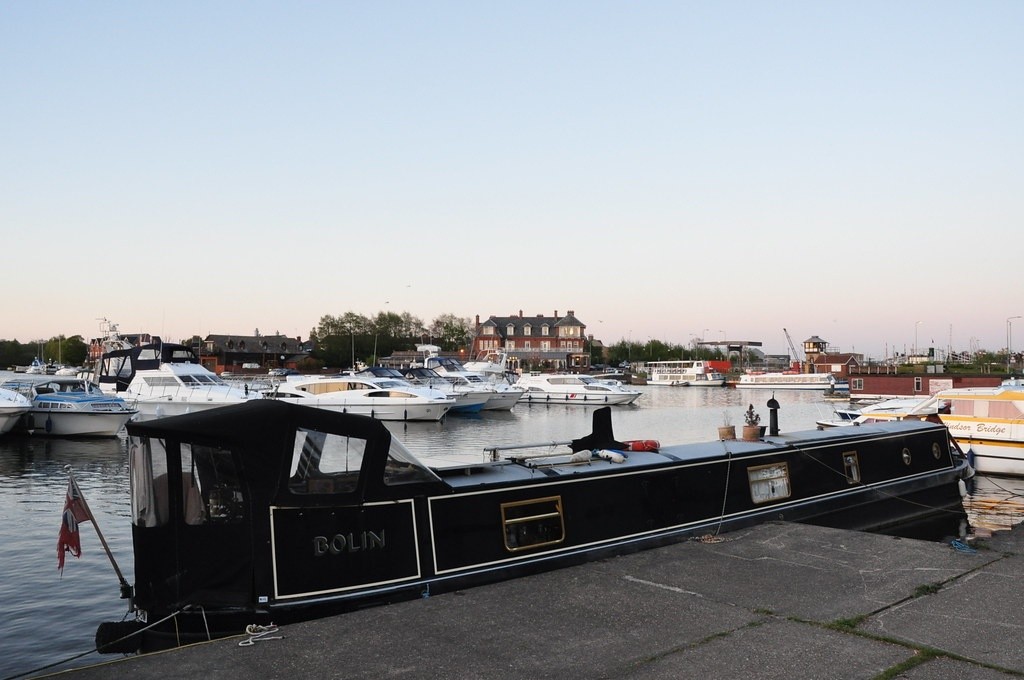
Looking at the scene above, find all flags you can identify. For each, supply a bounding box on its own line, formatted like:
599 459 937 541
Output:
56 474 92 580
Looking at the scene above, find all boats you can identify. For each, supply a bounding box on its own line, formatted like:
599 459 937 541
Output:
93 368 977 657
0 330 1024 481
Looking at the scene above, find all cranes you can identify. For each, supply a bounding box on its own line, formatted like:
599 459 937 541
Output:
782 328 807 373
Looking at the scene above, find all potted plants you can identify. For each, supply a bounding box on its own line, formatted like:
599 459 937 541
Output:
742 405 760 442
718 410 738 440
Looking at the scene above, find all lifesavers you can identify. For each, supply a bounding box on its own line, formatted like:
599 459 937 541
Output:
622 439 661 451
